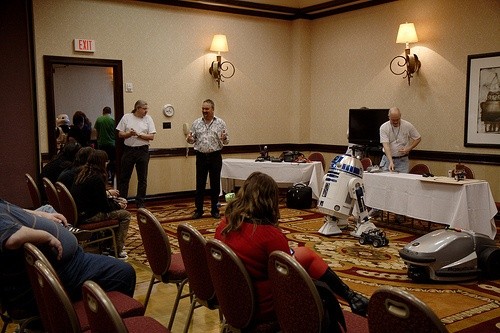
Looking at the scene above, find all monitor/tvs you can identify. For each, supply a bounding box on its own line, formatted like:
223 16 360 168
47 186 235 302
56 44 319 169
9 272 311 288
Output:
348 109 390 148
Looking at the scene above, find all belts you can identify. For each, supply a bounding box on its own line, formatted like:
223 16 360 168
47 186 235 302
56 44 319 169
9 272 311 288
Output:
385 153 408 159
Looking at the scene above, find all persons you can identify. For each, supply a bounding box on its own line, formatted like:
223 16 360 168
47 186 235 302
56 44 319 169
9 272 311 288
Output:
69 149 131 260
116 99 156 209
367 107 421 223
187 99 230 219
93 106 115 190
57 146 120 197
0 197 136 301
214 170 370 333
38 142 82 205
56 110 94 147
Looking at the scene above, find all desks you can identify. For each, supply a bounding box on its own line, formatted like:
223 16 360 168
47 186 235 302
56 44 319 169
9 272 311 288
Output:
219 158 324 208
362 170 498 240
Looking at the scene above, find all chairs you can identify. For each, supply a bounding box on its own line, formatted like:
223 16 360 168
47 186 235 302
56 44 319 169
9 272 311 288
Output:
308 153 326 171
360 157 474 180
0 174 450 333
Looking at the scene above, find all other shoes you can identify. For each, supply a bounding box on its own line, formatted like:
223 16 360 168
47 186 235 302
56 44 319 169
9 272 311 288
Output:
108 251 128 260
102 248 112 254
136 203 145 208
211 213 220 218
192 213 202 219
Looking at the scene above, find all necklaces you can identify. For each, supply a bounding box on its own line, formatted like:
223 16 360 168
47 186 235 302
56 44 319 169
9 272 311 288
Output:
391 121 400 143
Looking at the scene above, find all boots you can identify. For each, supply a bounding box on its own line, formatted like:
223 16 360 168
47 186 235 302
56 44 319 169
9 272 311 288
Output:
318 266 369 317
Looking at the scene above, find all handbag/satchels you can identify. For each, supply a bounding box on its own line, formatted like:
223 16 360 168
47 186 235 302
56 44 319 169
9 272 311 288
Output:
286 184 312 209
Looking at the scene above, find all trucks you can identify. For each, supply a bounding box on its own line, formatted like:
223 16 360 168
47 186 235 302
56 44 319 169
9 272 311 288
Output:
359 228 389 246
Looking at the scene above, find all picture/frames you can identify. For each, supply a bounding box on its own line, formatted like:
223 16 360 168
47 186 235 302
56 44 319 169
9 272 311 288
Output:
464 52 500 149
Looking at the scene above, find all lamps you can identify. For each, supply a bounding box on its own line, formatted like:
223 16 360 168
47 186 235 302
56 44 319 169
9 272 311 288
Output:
209 34 236 88
390 21 421 86
